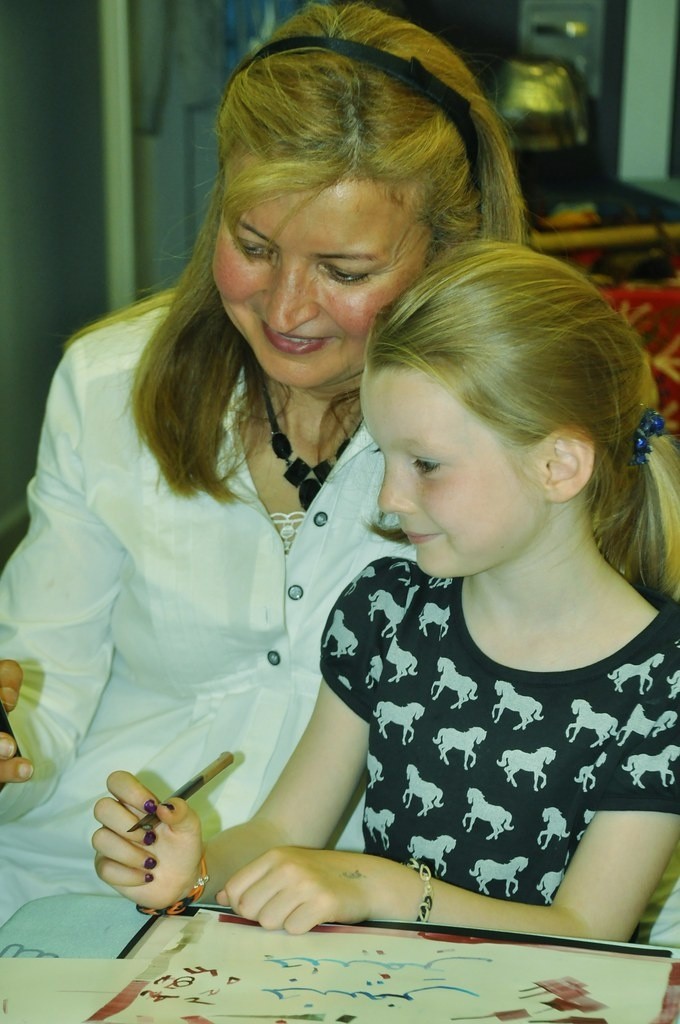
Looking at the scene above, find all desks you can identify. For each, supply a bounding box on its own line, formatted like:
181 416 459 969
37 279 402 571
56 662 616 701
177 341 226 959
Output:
0 893 680 1024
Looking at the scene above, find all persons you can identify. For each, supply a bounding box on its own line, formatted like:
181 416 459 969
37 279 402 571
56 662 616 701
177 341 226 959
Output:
0 4 680 954
92 238 680 943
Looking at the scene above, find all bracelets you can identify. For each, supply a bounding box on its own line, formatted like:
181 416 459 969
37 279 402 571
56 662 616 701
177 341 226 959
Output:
414 861 433 924
135 850 209 918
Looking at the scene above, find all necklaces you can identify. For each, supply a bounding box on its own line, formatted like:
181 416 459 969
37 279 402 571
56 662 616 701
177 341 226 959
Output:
260 379 366 512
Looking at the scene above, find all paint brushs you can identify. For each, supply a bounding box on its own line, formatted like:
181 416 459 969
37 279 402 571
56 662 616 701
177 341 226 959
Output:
125 749 234 834
